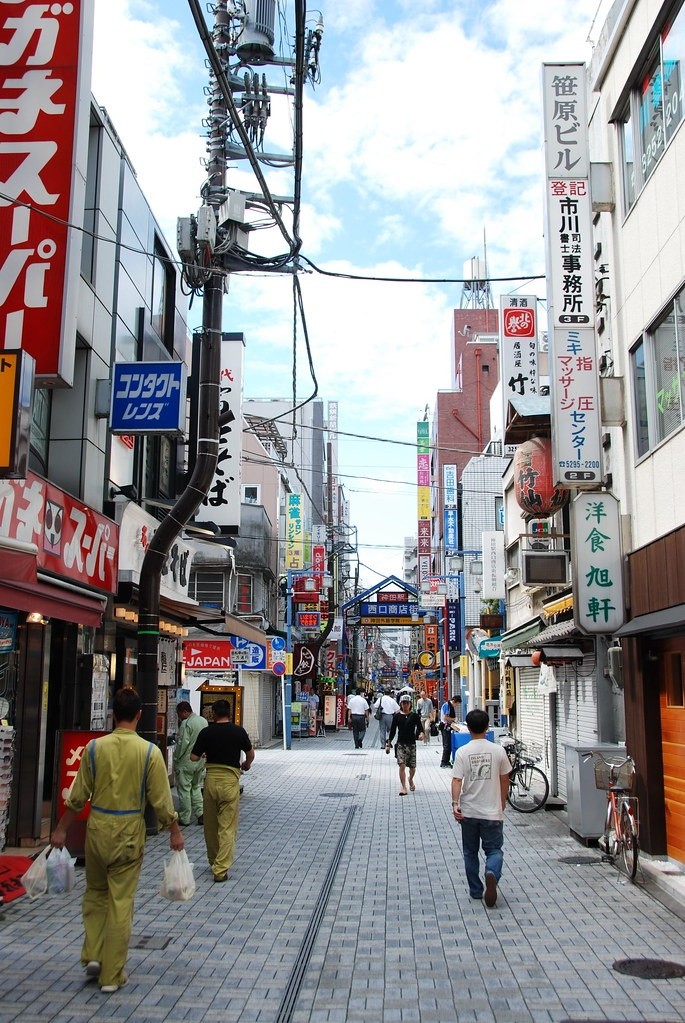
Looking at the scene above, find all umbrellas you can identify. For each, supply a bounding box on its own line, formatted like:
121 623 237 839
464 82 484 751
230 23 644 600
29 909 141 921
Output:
397 686 416 692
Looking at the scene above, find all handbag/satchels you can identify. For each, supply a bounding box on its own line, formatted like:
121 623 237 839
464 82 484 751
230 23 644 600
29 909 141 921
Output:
430 721 439 736
374 697 383 720
45 845 77 899
20 843 52 901
162 849 195 901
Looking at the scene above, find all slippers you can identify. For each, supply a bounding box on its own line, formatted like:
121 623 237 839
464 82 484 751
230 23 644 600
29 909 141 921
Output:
399 791 406 796
409 780 415 791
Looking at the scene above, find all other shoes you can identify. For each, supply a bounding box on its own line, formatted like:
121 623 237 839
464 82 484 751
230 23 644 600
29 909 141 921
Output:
82 961 101 977
484 870 497 907
213 872 228 882
385 739 393 748
177 819 190 827
440 764 453 769
381 747 385 749
358 739 362 748
197 814 204 826
423 741 430 746
470 894 483 899
355 747 359 748
101 979 128 992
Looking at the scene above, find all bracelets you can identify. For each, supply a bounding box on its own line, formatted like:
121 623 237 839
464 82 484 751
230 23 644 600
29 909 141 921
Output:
451 800 458 806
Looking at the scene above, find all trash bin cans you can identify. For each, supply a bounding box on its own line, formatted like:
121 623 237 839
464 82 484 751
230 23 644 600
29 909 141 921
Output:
451 730 495 764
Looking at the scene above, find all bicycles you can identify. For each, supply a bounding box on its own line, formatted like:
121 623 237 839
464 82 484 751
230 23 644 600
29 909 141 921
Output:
498 731 552 814
581 749 649 886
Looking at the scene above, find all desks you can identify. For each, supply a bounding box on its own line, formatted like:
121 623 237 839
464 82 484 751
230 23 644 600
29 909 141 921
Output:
451 730 494 764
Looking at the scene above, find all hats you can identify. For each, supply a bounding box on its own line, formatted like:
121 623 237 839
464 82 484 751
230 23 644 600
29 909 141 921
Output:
400 695 412 702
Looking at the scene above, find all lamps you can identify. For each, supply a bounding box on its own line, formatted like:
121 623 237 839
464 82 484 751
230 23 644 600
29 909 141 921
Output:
504 567 521 583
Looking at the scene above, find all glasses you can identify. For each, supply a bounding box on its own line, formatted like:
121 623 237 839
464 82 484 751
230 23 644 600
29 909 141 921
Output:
0 729 16 846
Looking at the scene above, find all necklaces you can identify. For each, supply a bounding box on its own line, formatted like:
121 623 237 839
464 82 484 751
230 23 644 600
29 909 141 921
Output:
404 711 409 718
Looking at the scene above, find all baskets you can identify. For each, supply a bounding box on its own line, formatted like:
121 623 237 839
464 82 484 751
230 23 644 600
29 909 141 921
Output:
593 759 633 790
519 739 543 762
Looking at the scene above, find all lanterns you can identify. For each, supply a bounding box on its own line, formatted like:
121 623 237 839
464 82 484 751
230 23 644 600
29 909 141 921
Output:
532 650 552 667
514 435 569 537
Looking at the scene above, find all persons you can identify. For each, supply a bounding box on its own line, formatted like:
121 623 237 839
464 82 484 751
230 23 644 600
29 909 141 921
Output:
415 690 435 746
403 691 407 695
386 695 425 795
345 690 400 749
173 700 204 827
371 687 403 705
451 708 513 907
307 687 319 711
431 689 462 767
190 701 255 883
51 687 185 992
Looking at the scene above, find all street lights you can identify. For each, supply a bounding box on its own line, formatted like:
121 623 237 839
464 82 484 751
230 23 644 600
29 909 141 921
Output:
449 549 507 726
282 569 332 751
